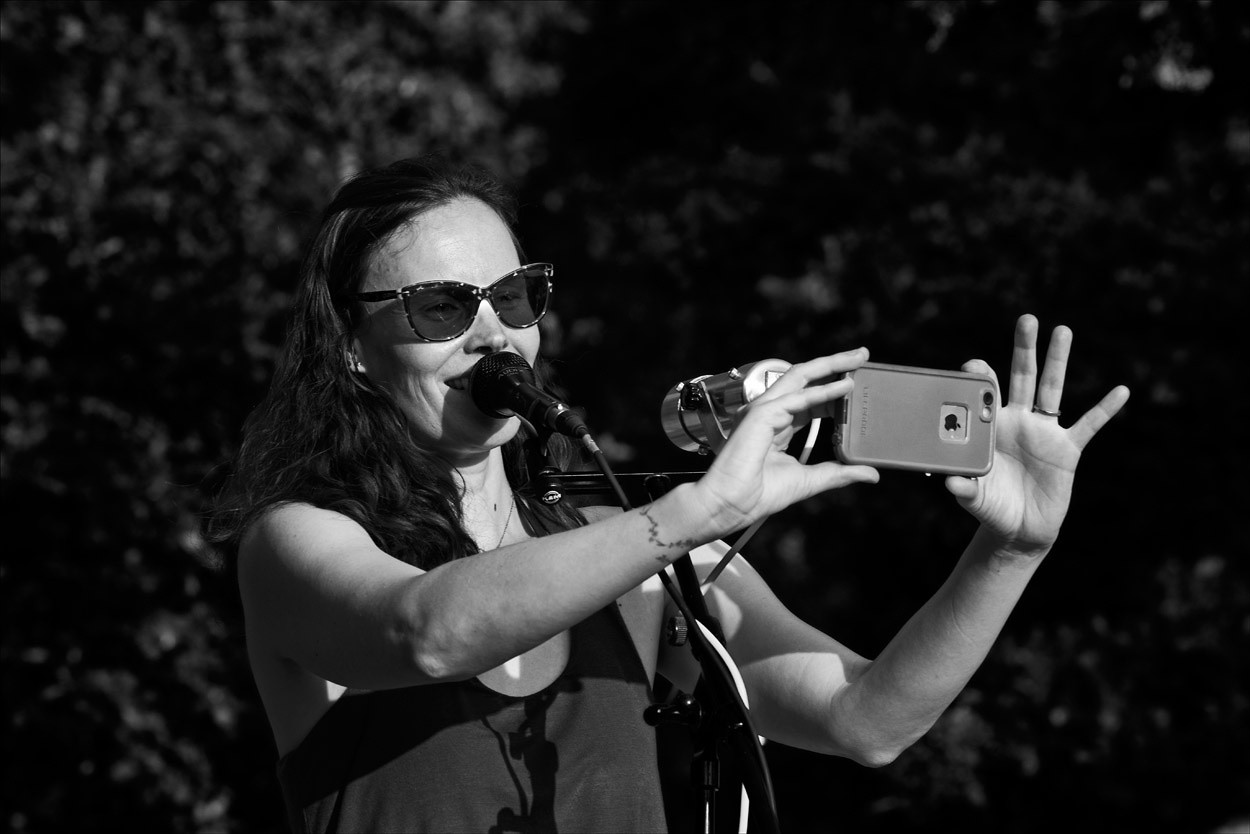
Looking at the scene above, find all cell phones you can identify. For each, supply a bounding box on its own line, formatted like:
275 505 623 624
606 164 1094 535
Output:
833 361 997 477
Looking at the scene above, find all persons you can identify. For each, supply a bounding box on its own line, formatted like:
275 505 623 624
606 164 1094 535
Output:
196 157 1134 833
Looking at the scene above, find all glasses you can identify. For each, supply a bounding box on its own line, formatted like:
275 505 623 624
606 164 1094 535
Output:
340 263 552 342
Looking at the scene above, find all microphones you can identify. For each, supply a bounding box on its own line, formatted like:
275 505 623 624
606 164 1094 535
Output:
661 359 795 452
469 351 589 437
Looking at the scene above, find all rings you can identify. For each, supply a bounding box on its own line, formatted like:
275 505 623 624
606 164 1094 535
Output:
1032 406 1062 417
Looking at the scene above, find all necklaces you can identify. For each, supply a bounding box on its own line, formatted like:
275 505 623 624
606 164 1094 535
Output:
477 492 516 553
